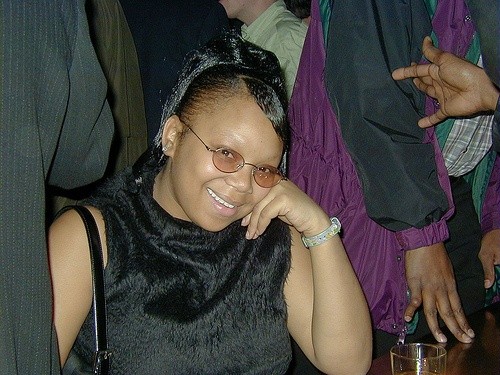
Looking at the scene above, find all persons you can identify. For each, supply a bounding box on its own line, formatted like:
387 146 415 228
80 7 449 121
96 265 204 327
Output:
46 29 375 375
216 0 312 104
46 0 149 228
0 0 116 375
285 0 500 344
390 35 500 155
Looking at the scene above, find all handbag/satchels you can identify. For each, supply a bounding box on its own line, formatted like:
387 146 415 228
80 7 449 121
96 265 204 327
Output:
54 205 112 375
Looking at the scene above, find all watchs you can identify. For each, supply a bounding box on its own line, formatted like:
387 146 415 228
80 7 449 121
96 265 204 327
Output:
301 216 342 249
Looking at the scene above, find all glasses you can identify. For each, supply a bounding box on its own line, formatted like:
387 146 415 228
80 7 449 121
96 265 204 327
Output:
181 121 283 188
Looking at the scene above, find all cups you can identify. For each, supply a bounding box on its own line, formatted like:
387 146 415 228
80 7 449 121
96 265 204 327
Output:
390 343 447 375
